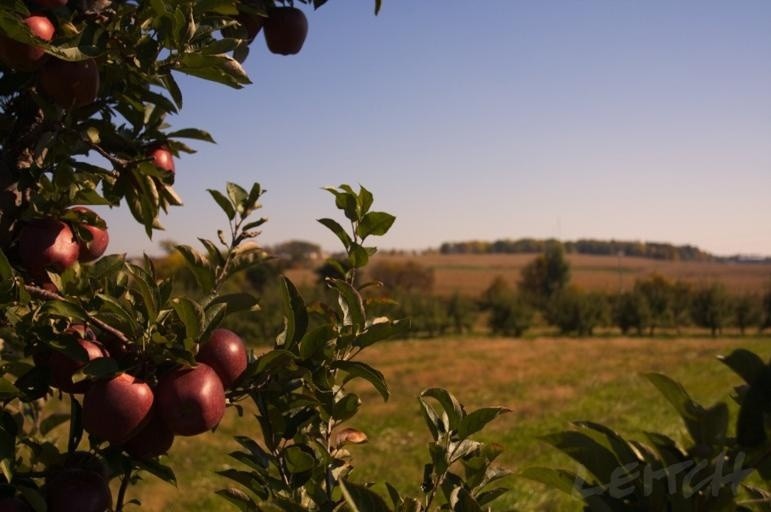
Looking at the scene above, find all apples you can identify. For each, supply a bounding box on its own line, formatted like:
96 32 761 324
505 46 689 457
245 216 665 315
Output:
15 14 246 439
